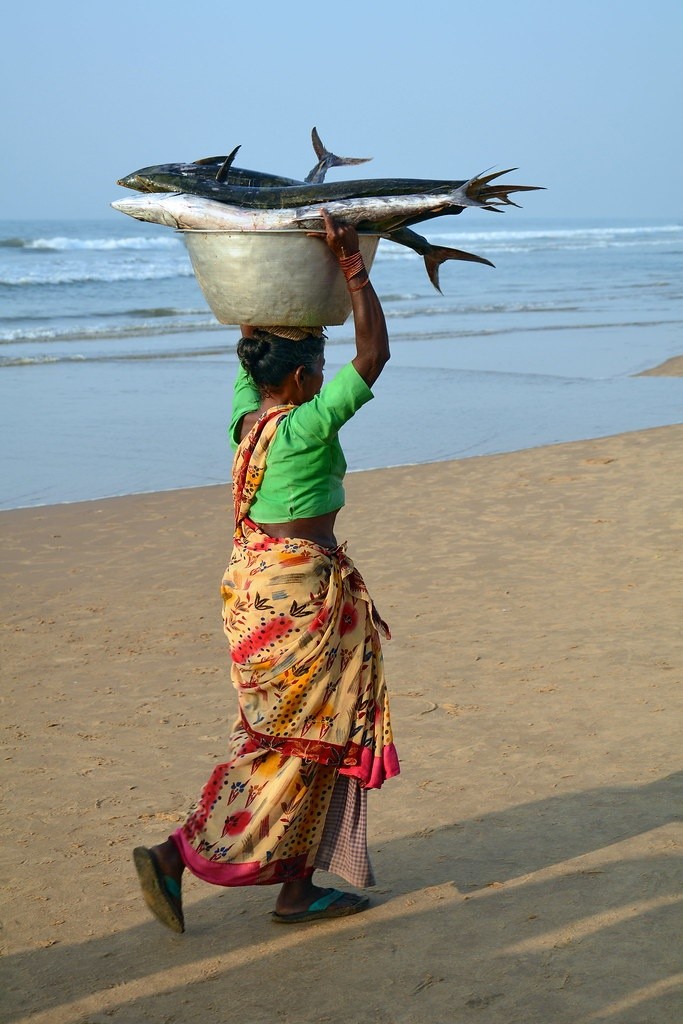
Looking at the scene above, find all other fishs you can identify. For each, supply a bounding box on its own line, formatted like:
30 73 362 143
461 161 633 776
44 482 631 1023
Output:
109 127 548 299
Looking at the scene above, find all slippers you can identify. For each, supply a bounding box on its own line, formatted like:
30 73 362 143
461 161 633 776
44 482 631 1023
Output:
271 885 370 923
133 845 186 935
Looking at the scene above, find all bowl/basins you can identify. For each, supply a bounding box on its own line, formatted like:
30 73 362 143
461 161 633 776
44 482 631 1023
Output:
174 218 392 331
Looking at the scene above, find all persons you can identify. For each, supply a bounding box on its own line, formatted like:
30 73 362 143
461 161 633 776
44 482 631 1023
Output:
132 208 399 934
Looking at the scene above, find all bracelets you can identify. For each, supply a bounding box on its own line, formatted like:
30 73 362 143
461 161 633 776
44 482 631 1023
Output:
337 248 373 291
